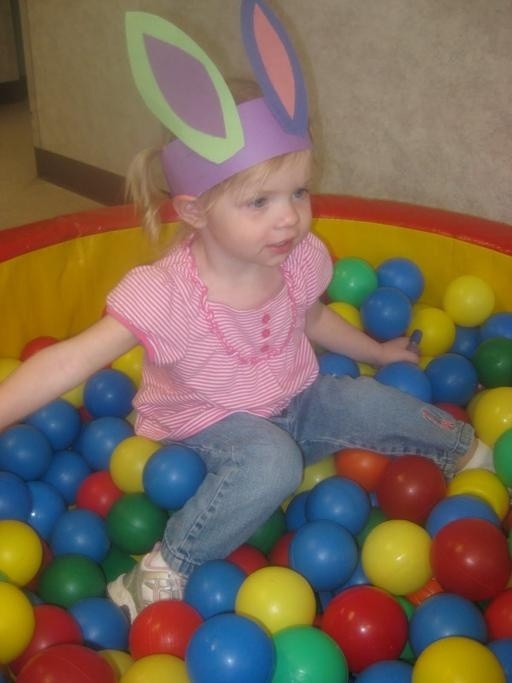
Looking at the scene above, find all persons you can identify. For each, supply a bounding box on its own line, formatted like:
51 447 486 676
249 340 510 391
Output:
0 75 497 627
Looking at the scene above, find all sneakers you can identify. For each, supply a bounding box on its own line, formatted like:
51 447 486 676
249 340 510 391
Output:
100 540 203 634
